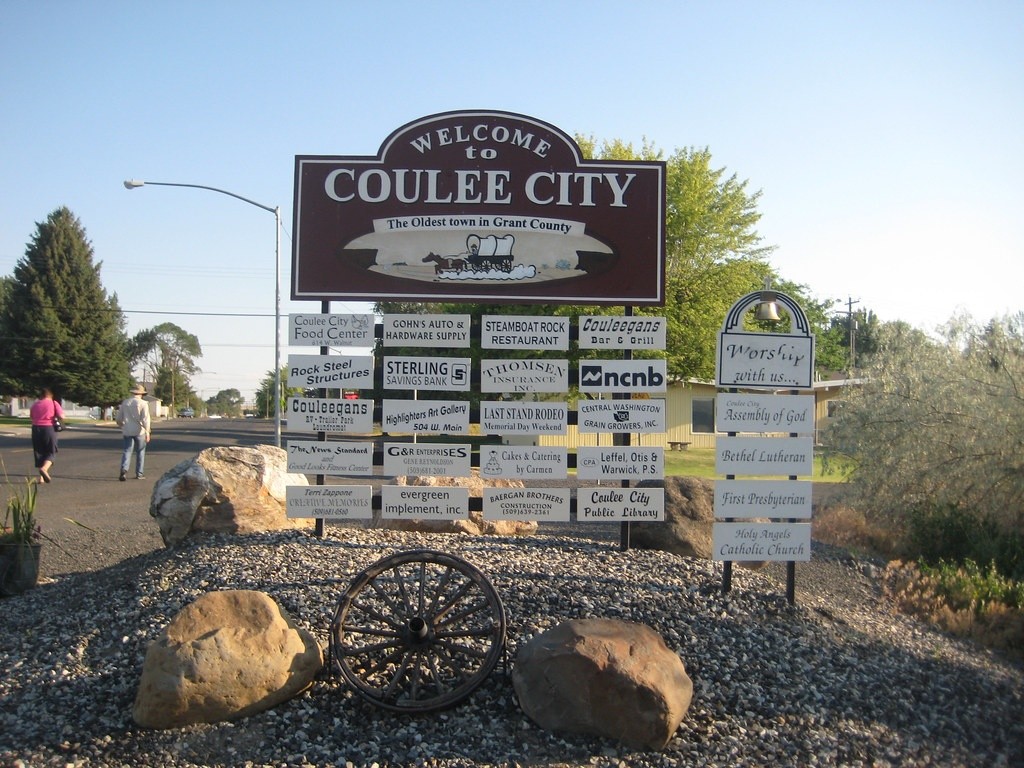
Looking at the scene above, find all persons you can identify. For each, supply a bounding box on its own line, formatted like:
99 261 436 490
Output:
116 384 150 480
29 387 65 484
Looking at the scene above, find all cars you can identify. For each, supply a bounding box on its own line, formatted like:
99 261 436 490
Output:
176 407 194 418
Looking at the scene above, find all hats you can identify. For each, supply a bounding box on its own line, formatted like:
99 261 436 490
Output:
129 383 147 394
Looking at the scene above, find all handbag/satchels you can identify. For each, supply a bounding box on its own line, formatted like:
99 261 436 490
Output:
53 400 65 430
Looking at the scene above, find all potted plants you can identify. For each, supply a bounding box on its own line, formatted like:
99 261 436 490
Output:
0 475 42 595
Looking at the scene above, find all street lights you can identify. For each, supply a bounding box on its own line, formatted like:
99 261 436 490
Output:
122 178 283 447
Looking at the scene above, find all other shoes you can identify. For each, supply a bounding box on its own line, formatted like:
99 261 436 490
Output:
40 469 50 483
136 475 145 479
119 469 127 481
